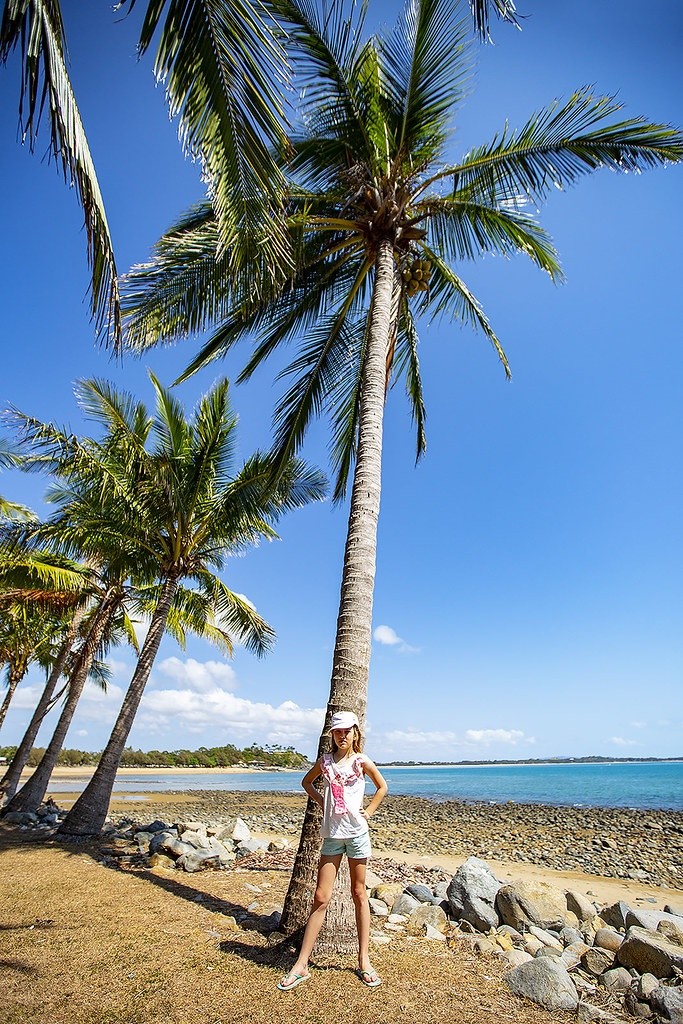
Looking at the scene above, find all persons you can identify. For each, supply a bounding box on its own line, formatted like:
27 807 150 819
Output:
276 710 388 991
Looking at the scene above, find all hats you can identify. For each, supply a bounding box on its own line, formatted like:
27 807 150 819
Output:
328 711 359 734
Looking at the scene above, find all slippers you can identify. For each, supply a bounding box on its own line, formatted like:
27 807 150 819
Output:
356 969 381 987
277 973 311 990
